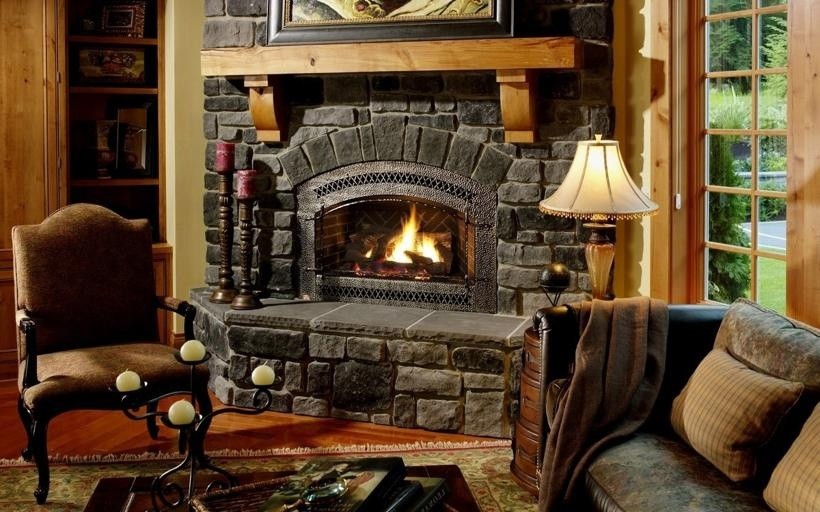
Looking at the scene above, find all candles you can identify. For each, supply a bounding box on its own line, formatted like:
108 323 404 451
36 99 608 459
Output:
167 397 197 425
237 169 256 199
179 339 207 361
252 358 277 386
115 369 142 392
215 142 234 175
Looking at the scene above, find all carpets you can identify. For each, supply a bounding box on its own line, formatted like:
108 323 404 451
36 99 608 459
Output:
0 438 540 512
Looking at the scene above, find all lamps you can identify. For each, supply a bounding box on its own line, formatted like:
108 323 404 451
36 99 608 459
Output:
538 128 660 300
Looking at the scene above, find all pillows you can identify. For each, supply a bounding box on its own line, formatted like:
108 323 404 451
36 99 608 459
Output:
671 344 805 481
714 298 820 403
763 402 819 512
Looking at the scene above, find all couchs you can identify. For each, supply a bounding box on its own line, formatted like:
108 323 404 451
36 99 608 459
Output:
509 304 773 511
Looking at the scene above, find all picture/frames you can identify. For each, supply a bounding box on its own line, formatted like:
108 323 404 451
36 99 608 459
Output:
266 1 514 46
97 0 147 39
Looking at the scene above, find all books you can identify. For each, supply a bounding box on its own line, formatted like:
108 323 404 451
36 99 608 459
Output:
259 456 454 512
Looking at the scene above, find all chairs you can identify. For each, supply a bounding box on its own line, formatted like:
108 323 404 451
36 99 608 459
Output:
11 203 211 505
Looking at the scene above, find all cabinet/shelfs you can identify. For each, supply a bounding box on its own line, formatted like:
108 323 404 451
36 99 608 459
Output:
66 0 166 244
